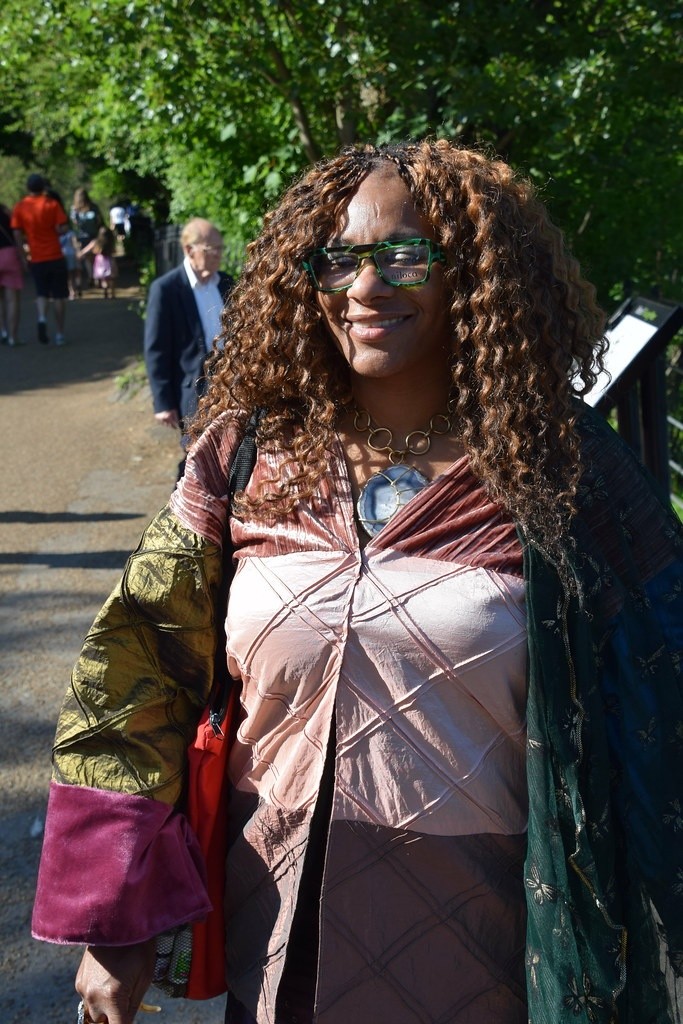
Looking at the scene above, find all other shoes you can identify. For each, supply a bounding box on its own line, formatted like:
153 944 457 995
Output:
56 334 65 345
1 331 7 338
9 338 15 346
37 318 49 345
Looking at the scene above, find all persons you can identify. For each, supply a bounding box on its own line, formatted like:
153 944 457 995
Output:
32 138 683 1024
144 216 236 493
0 174 139 347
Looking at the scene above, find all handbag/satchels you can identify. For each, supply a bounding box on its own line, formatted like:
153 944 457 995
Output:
146 413 260 1001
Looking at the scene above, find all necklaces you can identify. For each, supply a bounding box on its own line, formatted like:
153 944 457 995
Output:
341 391 459 537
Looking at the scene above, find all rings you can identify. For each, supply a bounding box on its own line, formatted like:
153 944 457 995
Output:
78 1000 107 1024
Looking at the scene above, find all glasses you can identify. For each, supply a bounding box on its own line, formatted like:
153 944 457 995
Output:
306 238 445 291
194 246 223 256
